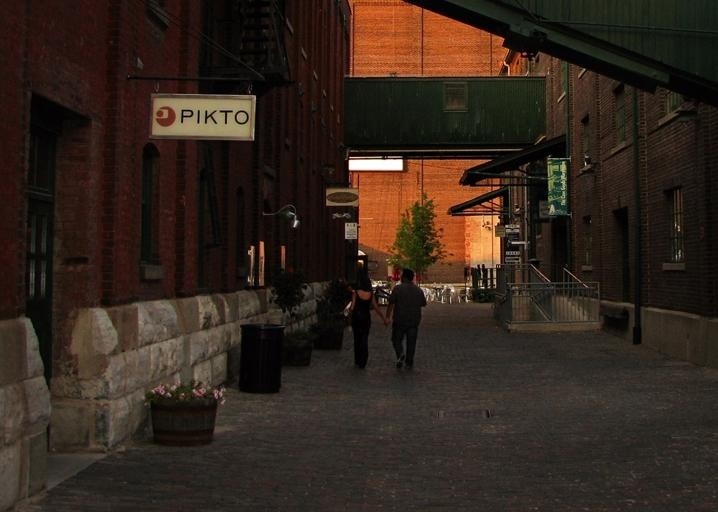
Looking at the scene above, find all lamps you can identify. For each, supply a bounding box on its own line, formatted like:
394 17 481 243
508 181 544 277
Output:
263 203 300 230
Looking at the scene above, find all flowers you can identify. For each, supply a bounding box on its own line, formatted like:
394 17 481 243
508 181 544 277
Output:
144 380 227 407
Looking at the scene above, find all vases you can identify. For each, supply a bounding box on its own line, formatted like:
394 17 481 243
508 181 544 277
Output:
150 400 218 446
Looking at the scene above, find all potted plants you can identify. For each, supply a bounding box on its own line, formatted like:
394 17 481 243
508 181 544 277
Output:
267 265 354 368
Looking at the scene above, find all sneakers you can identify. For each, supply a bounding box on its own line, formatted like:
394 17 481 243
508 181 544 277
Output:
396 354 412 370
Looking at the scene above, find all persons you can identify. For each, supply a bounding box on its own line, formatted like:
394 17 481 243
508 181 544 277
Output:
384 268 427 370
344 272 384 369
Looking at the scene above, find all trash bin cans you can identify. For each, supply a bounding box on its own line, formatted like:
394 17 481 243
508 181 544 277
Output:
238 323 285 394
